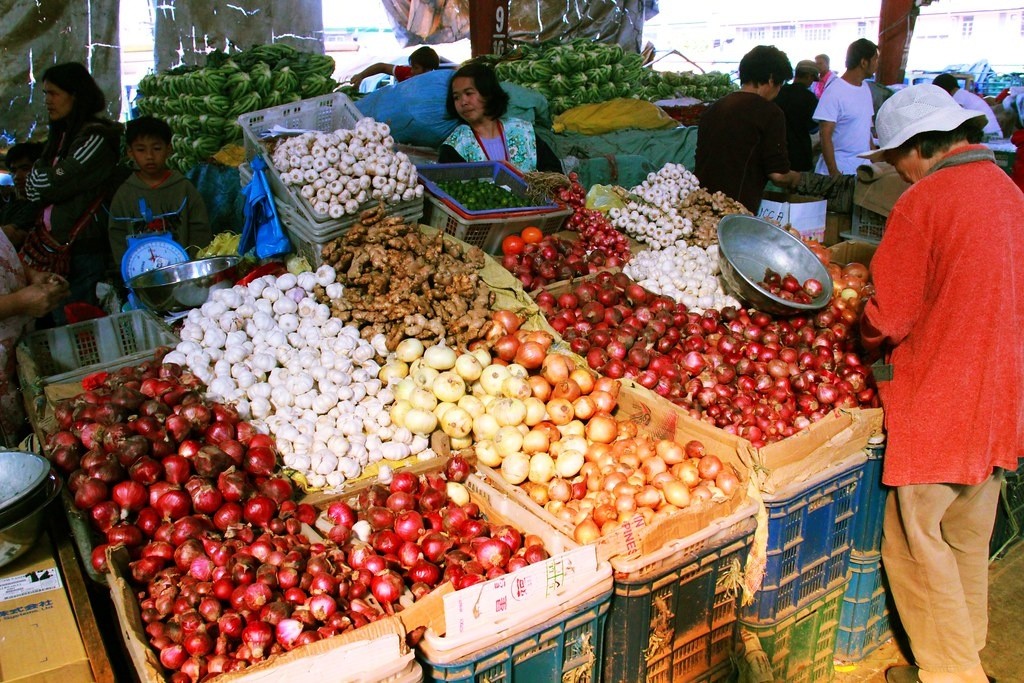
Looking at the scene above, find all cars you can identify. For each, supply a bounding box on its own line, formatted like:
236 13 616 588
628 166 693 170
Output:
381 55 460 86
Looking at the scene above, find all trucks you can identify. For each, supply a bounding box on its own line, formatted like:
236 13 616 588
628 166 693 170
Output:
910 61 1024 96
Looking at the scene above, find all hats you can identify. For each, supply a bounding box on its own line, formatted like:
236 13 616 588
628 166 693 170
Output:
796 60 820 82
856 83 988 164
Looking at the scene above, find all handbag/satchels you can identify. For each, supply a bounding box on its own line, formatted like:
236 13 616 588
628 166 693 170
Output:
16 218 70 278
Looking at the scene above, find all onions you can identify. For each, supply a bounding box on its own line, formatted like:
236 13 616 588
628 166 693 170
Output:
503 237 606 290
45 269 882 683
556 170 635 269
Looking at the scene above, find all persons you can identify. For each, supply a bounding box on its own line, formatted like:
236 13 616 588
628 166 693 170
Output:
812 38 879 175
439 64 565 175
856 85 1023 683
774 60 822 173
109 117 211 265
0 63 128 446
350 46 439 88
812 55 838 99
696 44 805 216
932 74 1003 140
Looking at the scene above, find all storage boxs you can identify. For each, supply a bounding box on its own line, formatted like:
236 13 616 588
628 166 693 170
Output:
0 90 898 683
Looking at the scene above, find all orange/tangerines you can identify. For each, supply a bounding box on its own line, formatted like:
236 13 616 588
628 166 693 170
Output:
437 176 535 211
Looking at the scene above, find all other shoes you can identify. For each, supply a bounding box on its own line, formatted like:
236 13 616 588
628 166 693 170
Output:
887 666 921 682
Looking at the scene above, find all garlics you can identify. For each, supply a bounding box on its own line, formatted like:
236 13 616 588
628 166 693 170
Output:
274 117 424 218
611 162 741 314
162 264 437 485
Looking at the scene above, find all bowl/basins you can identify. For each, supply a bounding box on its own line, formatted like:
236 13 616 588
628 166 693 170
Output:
122 254 244 316
717 213 834 318
0 450 64 568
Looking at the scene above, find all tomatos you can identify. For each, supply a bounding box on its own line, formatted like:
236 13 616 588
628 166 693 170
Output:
522 227 542 243
502 235 523 254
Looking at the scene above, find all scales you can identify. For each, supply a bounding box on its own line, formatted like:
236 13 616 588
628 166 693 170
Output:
100 196 193 327
